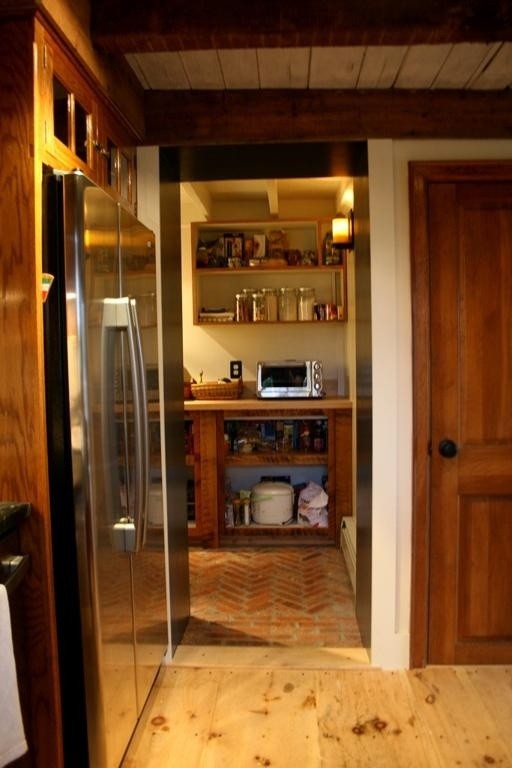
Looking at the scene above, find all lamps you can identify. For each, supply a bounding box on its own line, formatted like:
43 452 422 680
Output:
329 209 356 253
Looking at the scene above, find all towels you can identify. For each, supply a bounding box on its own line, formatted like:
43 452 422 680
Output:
0 585 30 768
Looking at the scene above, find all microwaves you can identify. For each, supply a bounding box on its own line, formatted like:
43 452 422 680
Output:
256 359 325 400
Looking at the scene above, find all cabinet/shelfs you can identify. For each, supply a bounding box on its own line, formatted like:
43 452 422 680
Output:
219 411 330 538
185 413 202 545
117 417 164 533
189 218 348 326
33 0 146 220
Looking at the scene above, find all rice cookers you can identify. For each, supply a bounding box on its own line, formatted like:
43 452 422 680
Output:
251 479 297 525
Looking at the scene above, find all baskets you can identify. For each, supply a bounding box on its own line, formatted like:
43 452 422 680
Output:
192 381 240 400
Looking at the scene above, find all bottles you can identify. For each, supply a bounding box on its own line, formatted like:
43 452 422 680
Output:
223 420 328 453
195 231 342 268
234 286 315 322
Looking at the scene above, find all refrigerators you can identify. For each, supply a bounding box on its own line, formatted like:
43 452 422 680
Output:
44 172 166 768
